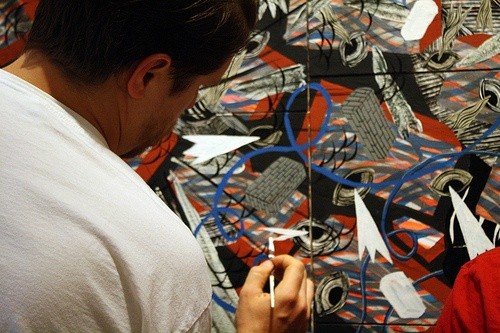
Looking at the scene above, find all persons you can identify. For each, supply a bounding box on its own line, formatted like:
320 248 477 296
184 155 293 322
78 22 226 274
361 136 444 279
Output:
0 0 316 333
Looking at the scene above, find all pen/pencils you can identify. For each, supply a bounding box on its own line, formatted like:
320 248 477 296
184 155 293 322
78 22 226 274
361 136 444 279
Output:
266 236 278 309
